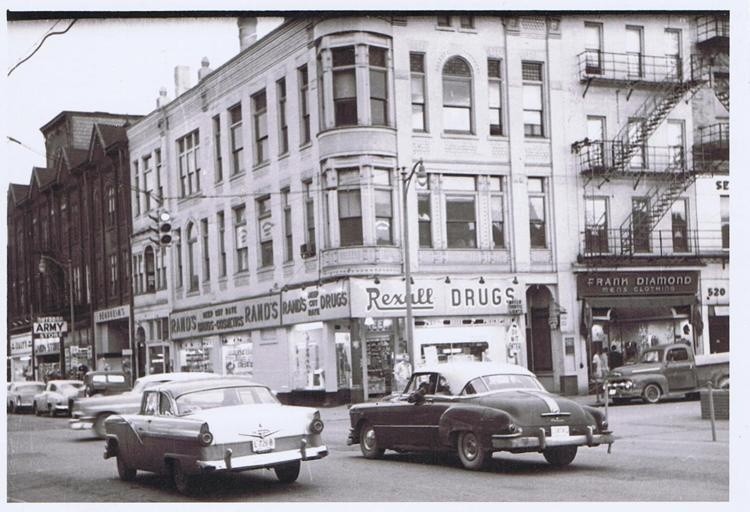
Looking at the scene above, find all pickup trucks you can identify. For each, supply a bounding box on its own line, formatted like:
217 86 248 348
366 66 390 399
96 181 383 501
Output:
603 342 729 405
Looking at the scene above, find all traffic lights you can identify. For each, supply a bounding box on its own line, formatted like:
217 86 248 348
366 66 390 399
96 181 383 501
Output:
158 208 173 247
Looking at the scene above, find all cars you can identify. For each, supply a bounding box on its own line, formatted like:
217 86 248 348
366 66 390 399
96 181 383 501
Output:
101 374 330 497
346 359 615 471
8 368 176 440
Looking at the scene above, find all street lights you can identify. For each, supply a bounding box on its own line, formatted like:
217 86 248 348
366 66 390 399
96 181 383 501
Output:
399 158 429 372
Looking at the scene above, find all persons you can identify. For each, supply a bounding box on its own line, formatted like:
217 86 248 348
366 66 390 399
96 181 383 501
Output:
592 345 624 377
